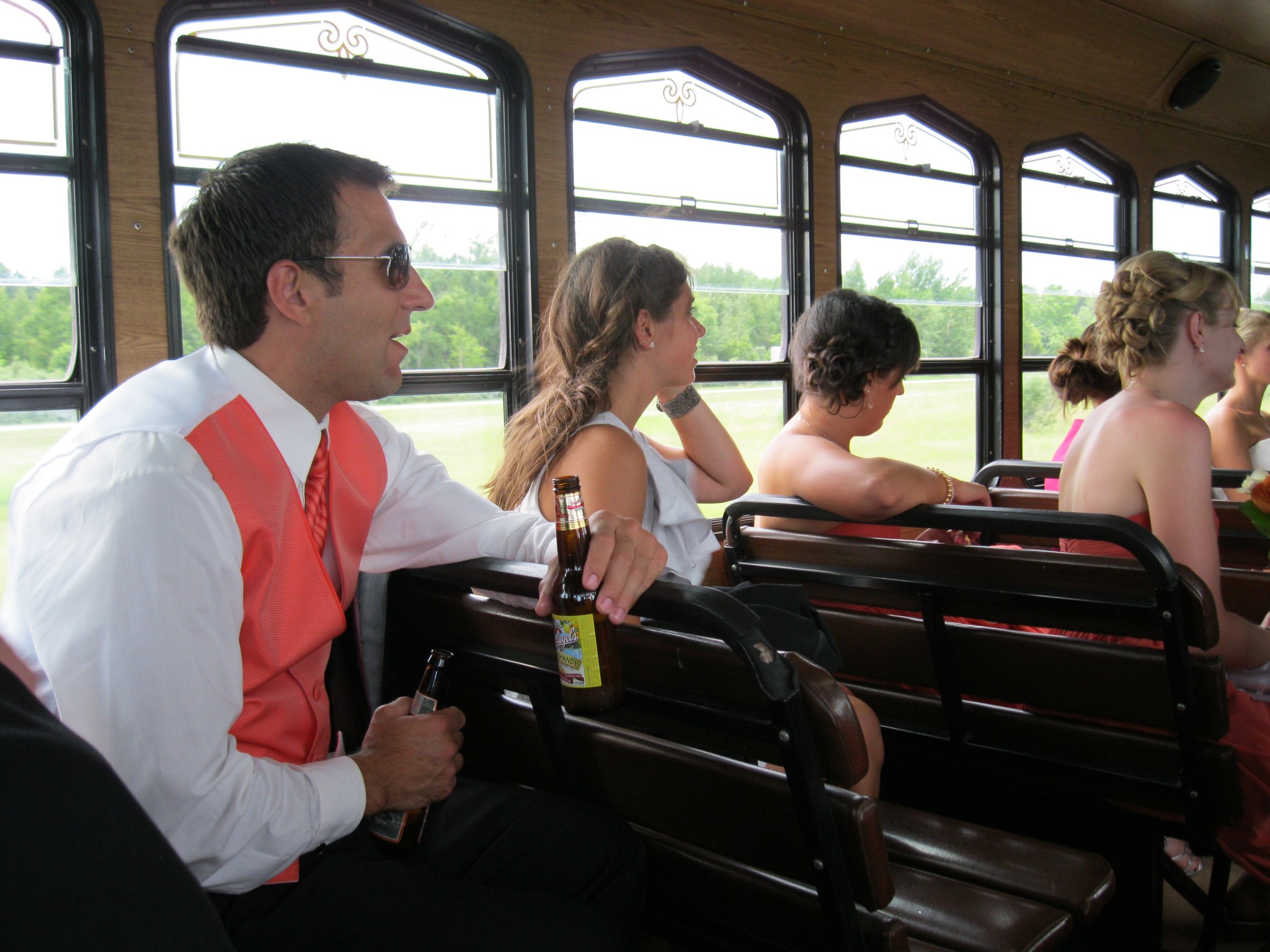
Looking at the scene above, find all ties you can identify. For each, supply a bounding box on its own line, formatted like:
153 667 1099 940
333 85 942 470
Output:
302 431 331 558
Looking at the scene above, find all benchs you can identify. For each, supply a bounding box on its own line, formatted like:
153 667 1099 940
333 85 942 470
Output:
381 458 1269 950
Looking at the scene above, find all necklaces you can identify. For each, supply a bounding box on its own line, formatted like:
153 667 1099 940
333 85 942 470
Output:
796 412 836 444
1127 382 1169 401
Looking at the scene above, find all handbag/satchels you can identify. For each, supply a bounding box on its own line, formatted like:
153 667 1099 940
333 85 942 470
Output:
639 580 844 676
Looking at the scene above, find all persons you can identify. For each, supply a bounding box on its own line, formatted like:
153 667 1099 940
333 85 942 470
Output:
0 136 673 949
482 234 902 918
1055 251 1270 888
1040 323 1122 491
1201 307 1270 502
755 288 1203 881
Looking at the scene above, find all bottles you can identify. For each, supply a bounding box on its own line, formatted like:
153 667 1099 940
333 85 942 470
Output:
547 472 632 720
369 643 461 848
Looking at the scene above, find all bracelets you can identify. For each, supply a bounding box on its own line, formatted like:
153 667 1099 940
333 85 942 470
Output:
947 475 955 505
657 384 702 419
926 466 952 505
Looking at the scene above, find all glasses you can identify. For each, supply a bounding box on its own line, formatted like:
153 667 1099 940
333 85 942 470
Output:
291 242 413 291
